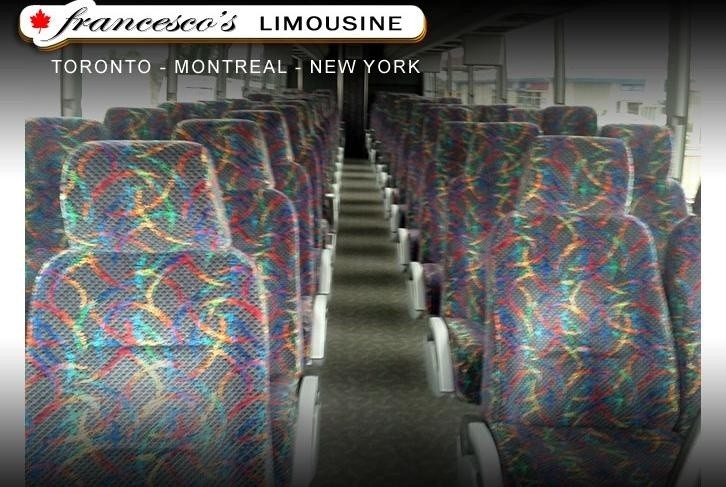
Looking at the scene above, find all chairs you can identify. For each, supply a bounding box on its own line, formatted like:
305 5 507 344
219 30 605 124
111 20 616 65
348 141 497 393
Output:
365 90 701 485
25 87 346 487
